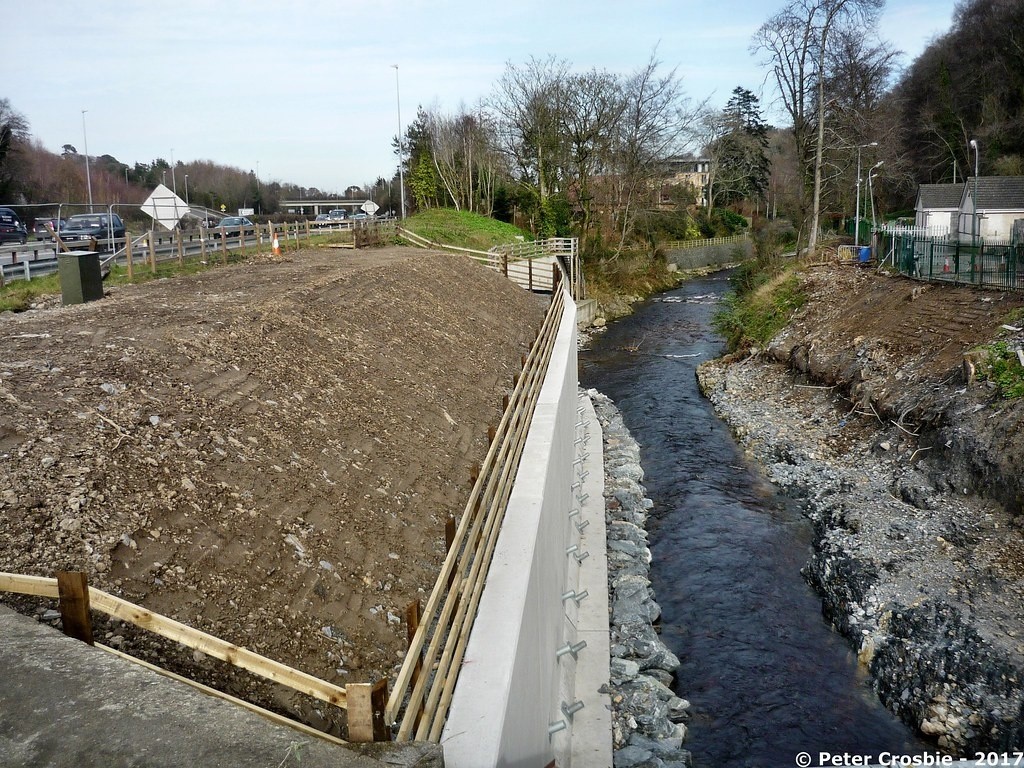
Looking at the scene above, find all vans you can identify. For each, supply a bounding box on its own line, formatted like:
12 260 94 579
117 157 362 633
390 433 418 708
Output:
0 208 28 245
33 218 66 241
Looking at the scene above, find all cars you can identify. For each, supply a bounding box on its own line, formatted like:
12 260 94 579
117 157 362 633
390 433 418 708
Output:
213 216 255 239
314 209 366 228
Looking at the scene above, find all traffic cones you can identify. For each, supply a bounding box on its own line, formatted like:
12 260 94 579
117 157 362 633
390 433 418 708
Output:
1000 254 1006 272
272 233 282 257
941 255 952 273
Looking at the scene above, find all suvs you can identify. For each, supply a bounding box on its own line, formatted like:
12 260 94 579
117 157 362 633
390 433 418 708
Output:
51 212 126 253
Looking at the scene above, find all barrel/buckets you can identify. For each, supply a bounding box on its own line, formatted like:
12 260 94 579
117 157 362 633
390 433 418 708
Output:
858 247 870 261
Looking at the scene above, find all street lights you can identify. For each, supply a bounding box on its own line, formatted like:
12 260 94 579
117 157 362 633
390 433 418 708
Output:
970 140 978 282
864 162 884 227
855 143 878 246
